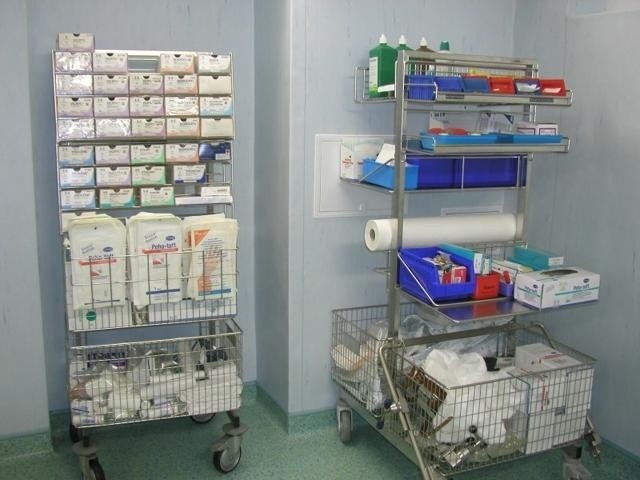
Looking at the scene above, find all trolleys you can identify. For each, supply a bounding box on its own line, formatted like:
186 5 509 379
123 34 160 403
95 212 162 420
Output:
331 51 603 480
50 50 249 479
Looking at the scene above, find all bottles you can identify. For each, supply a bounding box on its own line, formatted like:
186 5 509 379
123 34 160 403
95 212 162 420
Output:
415 37 435 74
369 34 399 97
396 36 414 76
437 40 452 76
